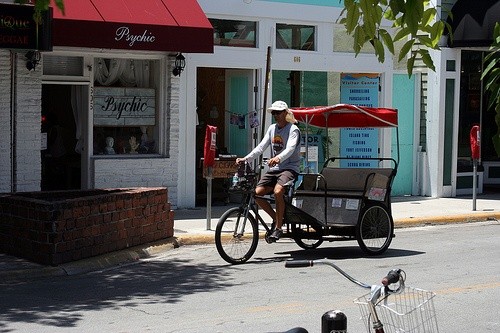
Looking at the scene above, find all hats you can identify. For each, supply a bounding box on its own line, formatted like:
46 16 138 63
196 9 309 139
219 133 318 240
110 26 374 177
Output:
267 101 288 113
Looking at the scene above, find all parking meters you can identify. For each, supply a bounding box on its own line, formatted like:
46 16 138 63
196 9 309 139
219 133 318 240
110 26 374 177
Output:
469 125 481 212
203 123 217 230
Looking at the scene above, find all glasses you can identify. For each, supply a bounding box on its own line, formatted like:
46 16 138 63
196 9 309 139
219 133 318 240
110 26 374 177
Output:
271 110 284 115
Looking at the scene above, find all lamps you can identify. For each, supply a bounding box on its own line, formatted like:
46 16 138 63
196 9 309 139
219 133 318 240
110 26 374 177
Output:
25 50 41 72
173 53 186 77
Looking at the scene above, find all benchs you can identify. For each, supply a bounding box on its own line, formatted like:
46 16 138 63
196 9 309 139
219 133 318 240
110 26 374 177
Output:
294 159 397 198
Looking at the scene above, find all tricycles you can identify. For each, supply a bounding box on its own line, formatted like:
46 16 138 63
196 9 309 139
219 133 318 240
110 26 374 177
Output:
215 105 400 264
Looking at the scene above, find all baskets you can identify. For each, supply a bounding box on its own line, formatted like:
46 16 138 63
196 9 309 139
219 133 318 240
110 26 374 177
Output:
226 171 255 204
353 284 439 333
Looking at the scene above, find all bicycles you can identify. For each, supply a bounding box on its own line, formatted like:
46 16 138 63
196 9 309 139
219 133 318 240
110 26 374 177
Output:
269 258 439 333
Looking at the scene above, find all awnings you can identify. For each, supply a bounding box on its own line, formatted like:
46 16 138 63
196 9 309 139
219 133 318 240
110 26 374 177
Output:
25 0 215 53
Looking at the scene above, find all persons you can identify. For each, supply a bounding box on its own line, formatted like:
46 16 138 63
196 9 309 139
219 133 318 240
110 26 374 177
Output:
128 136 140 153
106 136 115 155
236 101 301 240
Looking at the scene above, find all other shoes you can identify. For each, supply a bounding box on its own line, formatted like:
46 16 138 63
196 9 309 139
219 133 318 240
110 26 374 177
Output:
270 228 283 239
272 218 285 230
